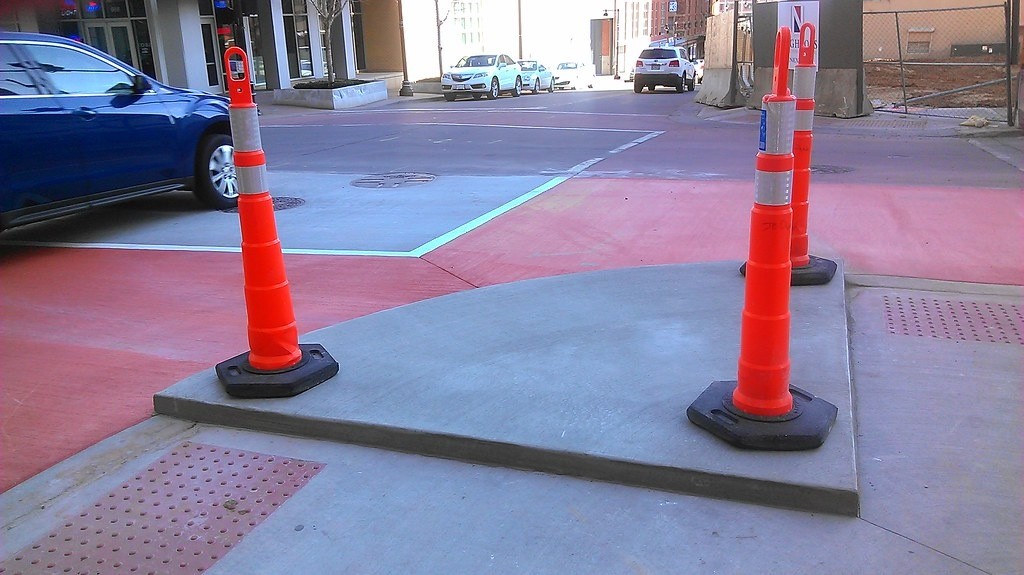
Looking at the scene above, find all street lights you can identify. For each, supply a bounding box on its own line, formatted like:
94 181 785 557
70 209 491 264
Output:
603 9 620 79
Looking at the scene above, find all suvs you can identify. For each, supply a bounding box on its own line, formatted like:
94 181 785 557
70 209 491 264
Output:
634 47 705 93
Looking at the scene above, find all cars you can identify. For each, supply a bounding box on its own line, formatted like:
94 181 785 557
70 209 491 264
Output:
517 61 555 95
0 31 239 230
441 54 523 101
554 62 597 91
